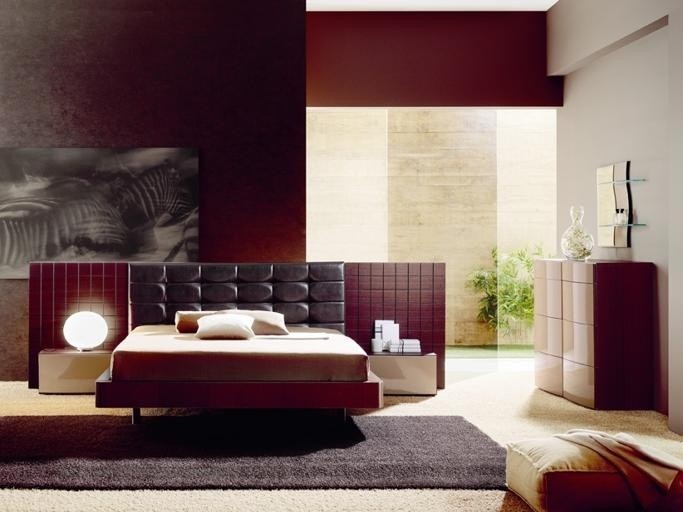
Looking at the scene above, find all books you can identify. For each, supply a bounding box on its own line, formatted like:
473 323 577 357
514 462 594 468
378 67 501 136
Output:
373 318 422 353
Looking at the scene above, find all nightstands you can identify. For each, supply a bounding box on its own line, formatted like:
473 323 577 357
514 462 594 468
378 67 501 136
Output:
358 342 438 396
37 349 112 395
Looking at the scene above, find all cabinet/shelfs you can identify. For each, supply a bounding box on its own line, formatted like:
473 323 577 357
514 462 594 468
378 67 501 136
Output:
532 256 653 410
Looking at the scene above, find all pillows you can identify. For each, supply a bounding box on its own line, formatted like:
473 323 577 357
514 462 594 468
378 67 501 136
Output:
175 309 290 339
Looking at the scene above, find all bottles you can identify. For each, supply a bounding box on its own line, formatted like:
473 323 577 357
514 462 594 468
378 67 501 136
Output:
612 208 621 225
620 208 627 225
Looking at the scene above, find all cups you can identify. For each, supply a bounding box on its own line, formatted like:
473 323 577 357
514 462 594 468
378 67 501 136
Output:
370 338 382 353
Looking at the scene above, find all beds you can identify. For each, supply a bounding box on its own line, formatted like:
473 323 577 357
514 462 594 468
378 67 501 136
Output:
94 262 384 425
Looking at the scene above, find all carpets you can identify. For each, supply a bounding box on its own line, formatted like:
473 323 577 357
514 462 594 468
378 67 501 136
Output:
0 415 507 491
